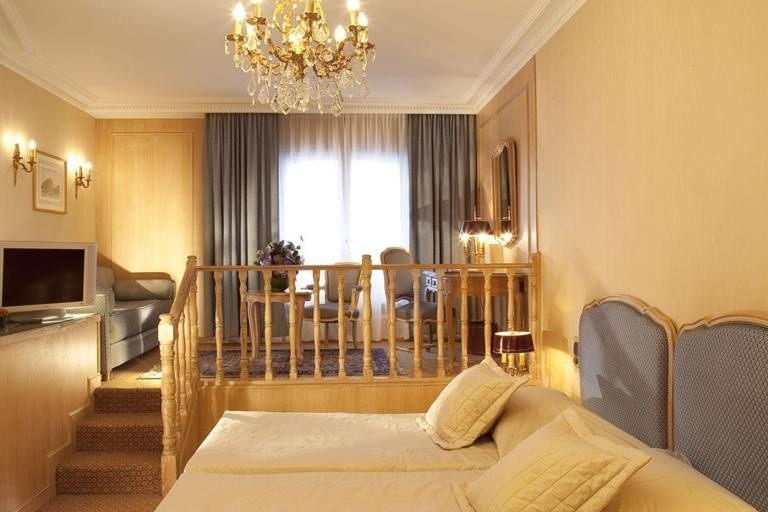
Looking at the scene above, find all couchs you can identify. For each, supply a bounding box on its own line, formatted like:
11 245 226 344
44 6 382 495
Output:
95 251 177 381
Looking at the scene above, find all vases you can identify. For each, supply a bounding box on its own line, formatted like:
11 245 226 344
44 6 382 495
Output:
253 237 302 289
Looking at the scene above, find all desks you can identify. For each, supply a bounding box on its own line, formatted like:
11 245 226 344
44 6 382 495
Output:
444 268 529 369
245 291 311 384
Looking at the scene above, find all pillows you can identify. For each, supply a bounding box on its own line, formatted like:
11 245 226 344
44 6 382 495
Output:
414 352 530 452
453 406 654 510
492 385 637 456
597 446 758 512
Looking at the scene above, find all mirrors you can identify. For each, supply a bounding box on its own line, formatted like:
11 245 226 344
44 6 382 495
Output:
490 137 520 249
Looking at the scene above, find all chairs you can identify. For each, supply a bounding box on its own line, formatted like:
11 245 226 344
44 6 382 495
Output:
303 262 360 350
382 245 456 351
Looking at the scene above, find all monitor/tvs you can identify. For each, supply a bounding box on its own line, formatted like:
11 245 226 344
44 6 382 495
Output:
0 241 98 324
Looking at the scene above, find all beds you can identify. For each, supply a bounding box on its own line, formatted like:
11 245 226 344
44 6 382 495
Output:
152 294 768 511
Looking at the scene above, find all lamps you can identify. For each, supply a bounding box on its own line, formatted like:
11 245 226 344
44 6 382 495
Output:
226 0 378 113
491 329 536 378
12 141 34 186
457 221 502 263
73 156 92 197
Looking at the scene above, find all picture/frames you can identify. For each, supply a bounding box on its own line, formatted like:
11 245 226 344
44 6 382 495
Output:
30 149 70 214
